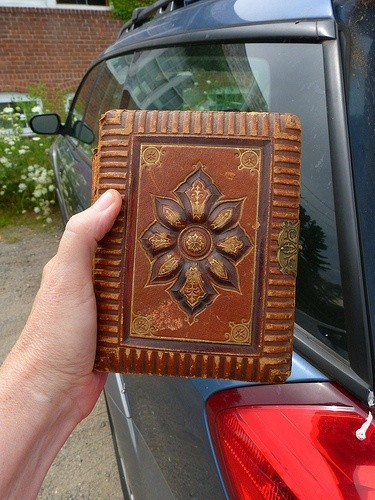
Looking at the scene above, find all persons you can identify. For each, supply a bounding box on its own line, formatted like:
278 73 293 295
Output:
0 188 122 500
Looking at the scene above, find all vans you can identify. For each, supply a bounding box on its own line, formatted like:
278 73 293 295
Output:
32 0 374 499
